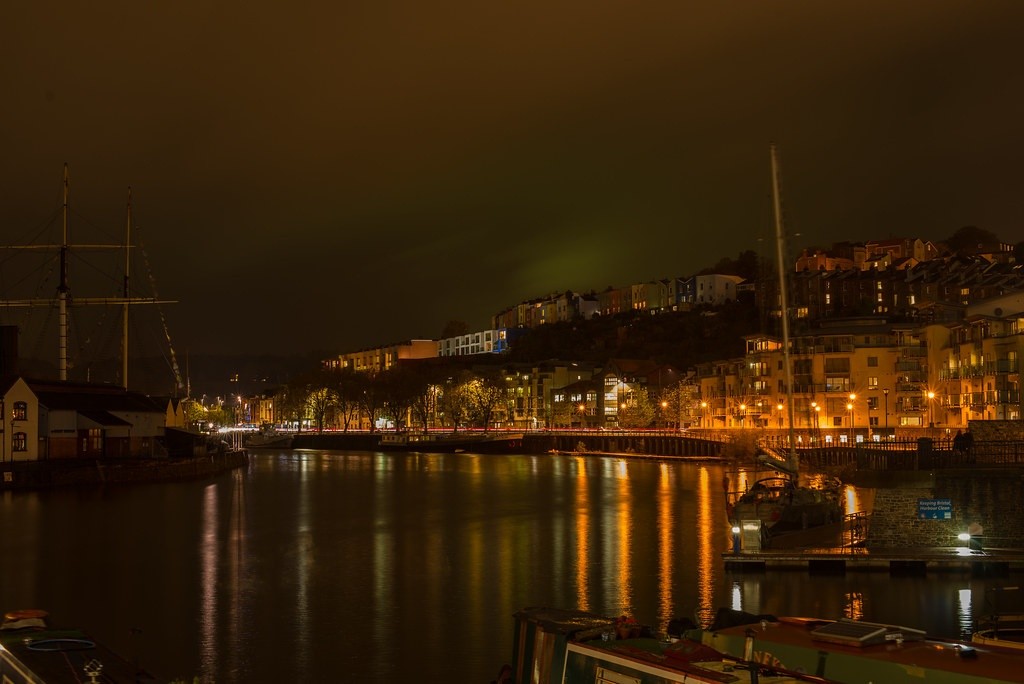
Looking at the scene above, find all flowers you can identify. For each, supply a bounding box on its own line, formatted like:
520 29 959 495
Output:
617 616 639 627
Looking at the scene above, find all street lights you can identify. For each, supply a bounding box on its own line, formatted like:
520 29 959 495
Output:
815 406 820 449
702 402 706 429
882 388 890 451
928 392 935 439
200 393 225 409
237 395 242 448
847 403 852 447
740 404 745 428
811 402 816 449
849 393 856 447
622 403 626 435
777 404 783 448
662 402 667 432
579 405 584 435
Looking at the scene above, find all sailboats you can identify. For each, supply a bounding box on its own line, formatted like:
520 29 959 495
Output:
719 138 850 554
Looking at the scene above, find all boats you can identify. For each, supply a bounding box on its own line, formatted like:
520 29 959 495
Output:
243 419 295 449
664 601 1024 684
492 604 847 684
0 608 163 684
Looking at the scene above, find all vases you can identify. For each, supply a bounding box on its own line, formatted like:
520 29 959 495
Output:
619 628 629 640
